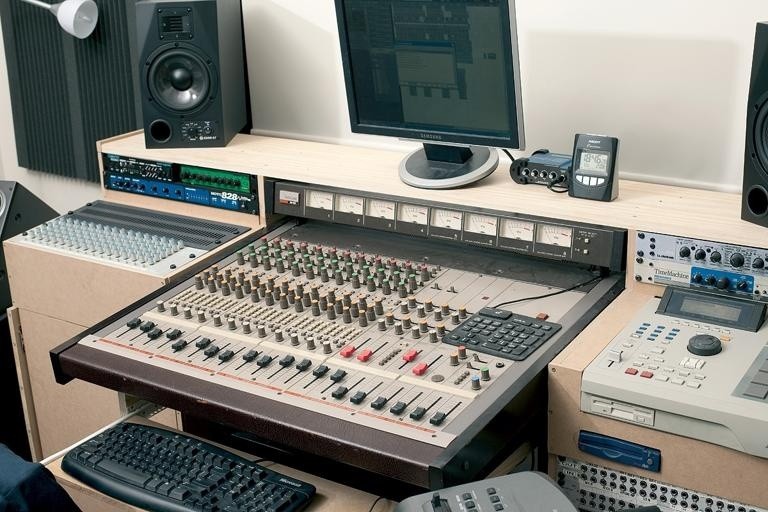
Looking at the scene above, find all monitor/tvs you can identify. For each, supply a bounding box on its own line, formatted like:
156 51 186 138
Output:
333 0 526 189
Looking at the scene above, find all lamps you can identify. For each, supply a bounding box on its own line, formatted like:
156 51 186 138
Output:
19 0 99 39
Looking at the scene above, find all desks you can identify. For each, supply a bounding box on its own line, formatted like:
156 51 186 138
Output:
2 128 768 512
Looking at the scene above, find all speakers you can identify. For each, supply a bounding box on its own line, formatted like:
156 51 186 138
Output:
740 20 768 229
134 0 248 149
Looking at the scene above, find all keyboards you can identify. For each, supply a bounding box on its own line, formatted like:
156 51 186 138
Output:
61 421 317 512
